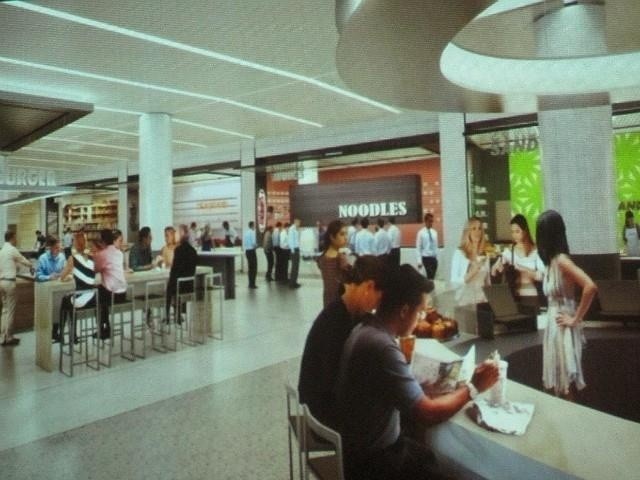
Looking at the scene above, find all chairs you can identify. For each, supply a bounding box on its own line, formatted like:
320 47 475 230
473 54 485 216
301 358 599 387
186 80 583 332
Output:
284 384 344 480
58 273 225 376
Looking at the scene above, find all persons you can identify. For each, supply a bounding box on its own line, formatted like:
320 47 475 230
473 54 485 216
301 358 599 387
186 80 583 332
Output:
622 211 640 256
33 221 238 344
244 213 439 289
492 213 546 306
536 209 597 401
1 231 33 346
298 254 501 479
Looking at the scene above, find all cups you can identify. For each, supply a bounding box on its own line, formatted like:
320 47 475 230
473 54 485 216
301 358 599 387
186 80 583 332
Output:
484 359 508 408
399 335 416 364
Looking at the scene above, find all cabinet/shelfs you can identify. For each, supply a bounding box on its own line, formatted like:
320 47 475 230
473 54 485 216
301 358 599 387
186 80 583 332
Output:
64 202 136 243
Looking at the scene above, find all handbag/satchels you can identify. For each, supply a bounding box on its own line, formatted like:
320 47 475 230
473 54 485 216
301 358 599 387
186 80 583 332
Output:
490 265 520 297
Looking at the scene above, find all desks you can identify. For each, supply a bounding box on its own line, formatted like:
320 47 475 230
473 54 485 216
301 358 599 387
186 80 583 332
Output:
34 265 215 372
198 250 239 299
